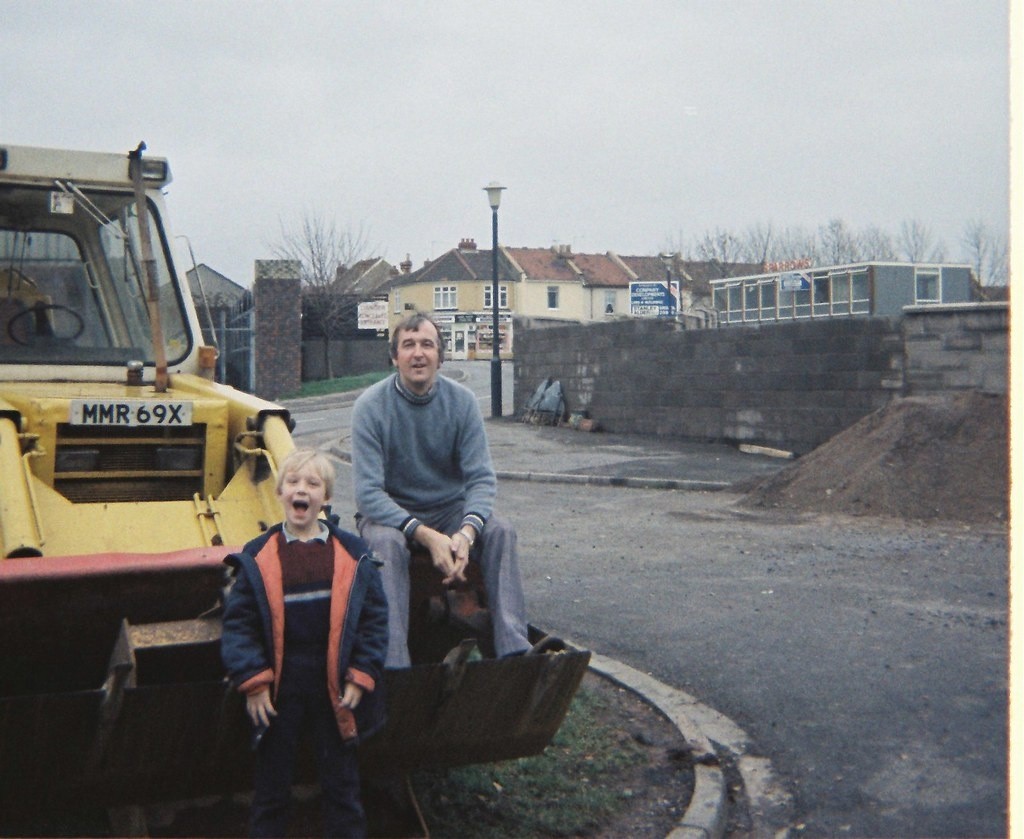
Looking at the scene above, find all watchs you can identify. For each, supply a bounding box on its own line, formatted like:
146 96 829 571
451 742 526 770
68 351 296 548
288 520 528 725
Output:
459 530 474 545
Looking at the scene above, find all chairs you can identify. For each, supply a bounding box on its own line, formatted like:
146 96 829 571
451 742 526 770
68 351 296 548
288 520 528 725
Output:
0 298 35 351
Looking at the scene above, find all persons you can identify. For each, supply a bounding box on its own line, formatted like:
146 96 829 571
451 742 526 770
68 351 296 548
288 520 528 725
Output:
351 310 531 675
218 447 393 839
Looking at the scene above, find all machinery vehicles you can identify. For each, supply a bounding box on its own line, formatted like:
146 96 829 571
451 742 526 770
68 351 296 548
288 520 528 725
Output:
0 142 591 839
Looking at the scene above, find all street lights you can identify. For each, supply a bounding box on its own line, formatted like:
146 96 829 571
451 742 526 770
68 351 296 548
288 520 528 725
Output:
483 178 507 417
661 254 675 320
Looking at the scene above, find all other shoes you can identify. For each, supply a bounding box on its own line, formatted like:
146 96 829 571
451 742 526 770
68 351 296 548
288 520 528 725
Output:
441 643 483 664
525 634 568 656
